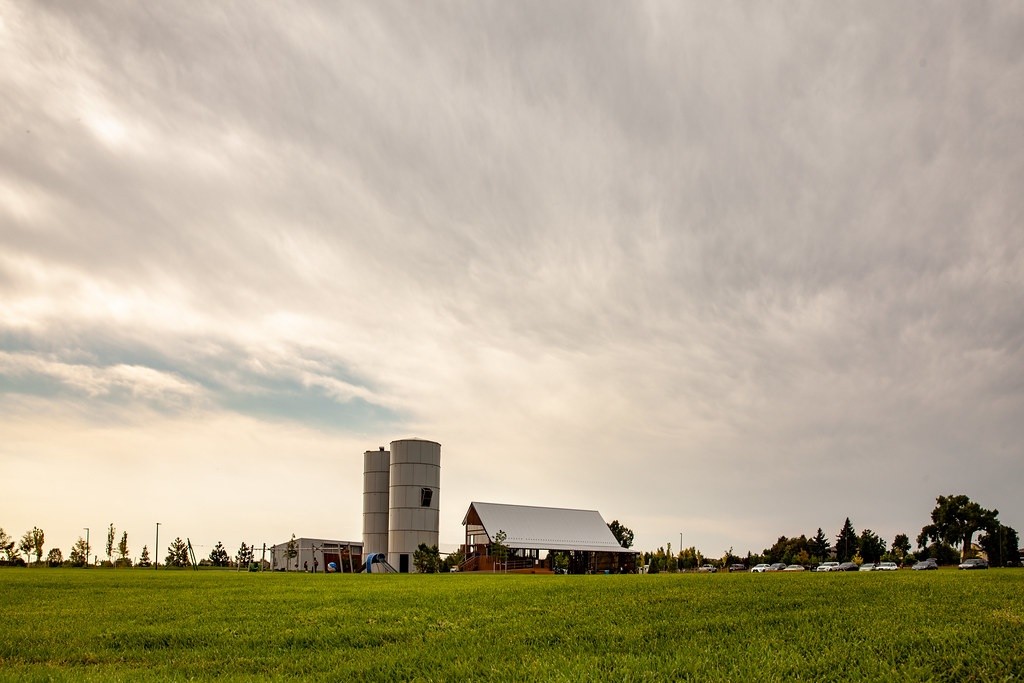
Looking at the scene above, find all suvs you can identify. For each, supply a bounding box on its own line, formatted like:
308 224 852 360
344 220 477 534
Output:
816 561 840 571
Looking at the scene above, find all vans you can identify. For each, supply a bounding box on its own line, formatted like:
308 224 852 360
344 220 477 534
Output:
875 561 897 571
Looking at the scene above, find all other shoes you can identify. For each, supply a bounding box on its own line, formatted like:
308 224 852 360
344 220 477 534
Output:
305 571 308 572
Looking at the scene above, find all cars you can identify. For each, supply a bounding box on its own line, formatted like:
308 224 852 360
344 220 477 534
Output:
836 562 858 572
958 558 989 570
730 564 747 571
783 564 805 571
859 562 878 571
769 563 787 571
750 563 770 573
699 564 718 573
912 562 939 571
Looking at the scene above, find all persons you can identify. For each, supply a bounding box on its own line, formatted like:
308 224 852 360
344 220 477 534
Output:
311 557 318 572
304 561 309 573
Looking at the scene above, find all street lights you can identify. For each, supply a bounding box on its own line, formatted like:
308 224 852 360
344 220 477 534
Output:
83 527 89 569
679 532 682 552
155 522 161 570
